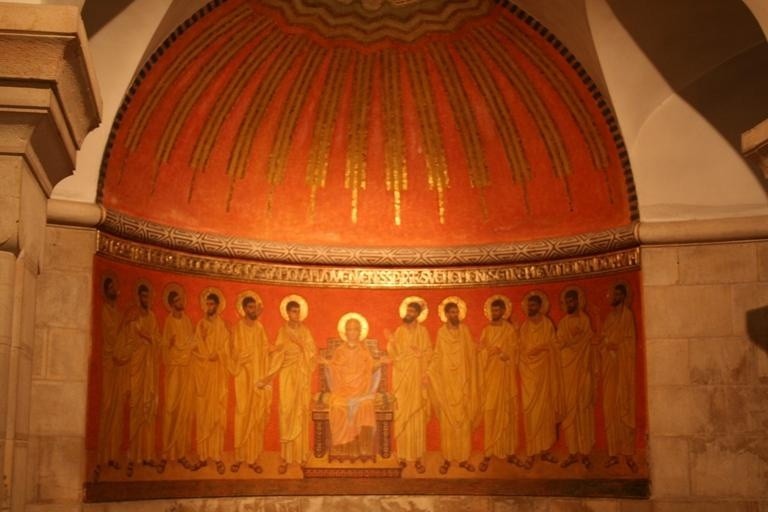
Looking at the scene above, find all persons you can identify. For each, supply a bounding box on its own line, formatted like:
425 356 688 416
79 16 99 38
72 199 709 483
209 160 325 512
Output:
112 284 162 480
190 295 230 474
517 294 561 469
478 299 520 470
95 278 124 477
556 290 601 470
598 284 641 473
272 302 316 473
156 290 194 472
225 296 285 474
386 302 433 473
316 318 394 445
426 302 482 474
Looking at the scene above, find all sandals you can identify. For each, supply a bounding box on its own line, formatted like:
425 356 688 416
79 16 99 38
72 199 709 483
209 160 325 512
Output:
401 457 490 473
178 456 262 473
91 460 166 481
507 452 639 473
279 461 288 473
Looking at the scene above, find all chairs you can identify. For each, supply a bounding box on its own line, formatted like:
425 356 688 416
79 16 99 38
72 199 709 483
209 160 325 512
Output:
301 338 406 476
